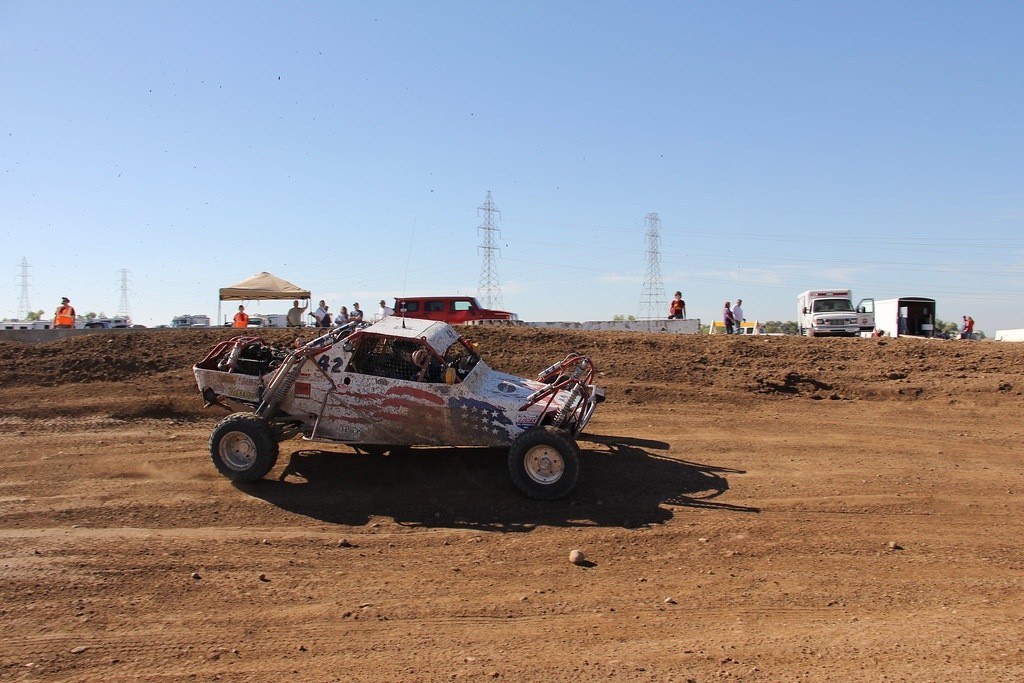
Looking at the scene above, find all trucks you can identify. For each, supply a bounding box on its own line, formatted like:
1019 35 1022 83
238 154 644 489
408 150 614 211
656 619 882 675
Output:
798 288 876 337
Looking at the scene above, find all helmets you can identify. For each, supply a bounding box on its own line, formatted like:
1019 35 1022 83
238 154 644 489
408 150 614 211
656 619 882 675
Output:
392 339 418 362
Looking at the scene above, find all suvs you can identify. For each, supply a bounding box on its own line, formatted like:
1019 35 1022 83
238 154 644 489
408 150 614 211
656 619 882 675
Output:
390 297 518 325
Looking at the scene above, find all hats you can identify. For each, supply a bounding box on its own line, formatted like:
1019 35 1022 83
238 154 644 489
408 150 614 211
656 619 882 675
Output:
353 302 358 306
379 300 386 304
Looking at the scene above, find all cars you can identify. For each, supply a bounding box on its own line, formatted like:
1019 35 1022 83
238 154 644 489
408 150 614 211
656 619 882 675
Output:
84 321 113 329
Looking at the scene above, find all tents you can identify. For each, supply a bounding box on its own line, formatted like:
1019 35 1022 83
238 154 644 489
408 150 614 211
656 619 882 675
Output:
219 271 312 328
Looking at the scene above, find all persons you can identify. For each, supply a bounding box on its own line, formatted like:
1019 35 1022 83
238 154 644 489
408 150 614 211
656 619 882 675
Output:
233 305 249 328
389 339 461 383
960 315 974 339
350 302 363 323
731 299 743 335
378 299 394 321
286 299 308 327
53 297 76 329
335 306 350 326
668 291 686 319
724 302 737 334
310 299 332 327
